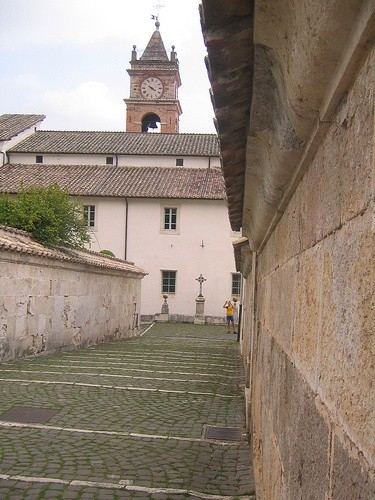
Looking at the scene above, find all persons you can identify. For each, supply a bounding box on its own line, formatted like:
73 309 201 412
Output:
222 300 238 335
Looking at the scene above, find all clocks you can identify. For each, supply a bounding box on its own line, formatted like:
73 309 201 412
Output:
139 76 165 103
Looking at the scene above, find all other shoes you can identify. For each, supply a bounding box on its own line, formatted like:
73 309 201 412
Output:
226 331 230 335
232 331 238 334
